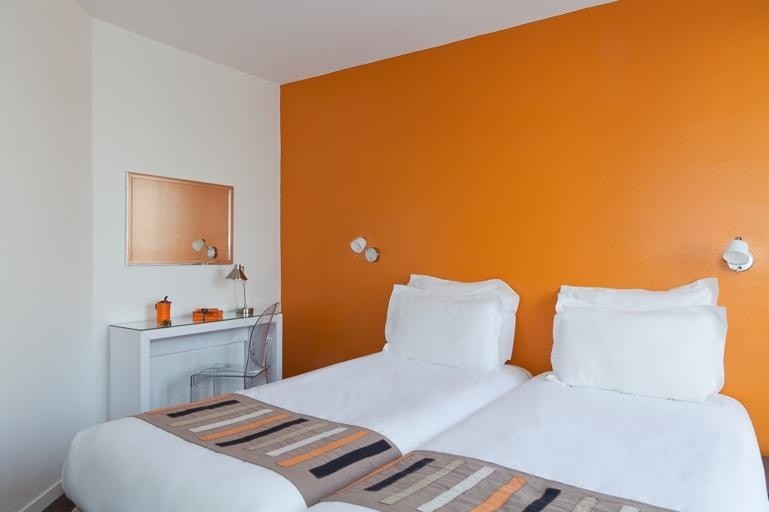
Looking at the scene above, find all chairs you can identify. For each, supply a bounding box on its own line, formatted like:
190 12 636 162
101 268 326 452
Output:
188 300 280 404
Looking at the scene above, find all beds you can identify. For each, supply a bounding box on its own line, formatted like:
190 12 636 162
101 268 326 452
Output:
56 273 535 511
306 274 769 512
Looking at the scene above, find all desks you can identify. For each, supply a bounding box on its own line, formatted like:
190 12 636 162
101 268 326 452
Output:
107 309 285 421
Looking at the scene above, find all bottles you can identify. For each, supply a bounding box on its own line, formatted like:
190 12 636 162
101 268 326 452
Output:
154 296 173 326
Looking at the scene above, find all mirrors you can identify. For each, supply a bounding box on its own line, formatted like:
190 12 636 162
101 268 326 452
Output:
124 169 234 267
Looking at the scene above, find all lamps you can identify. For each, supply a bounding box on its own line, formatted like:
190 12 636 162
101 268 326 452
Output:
349 235 381 264
719 234 757 273
191 238 218 259
225 262 254 315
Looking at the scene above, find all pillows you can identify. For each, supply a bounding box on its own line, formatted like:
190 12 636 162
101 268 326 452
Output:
558 275 721 308
381 282 508 371
406 270 520 363
545 293 730 405
